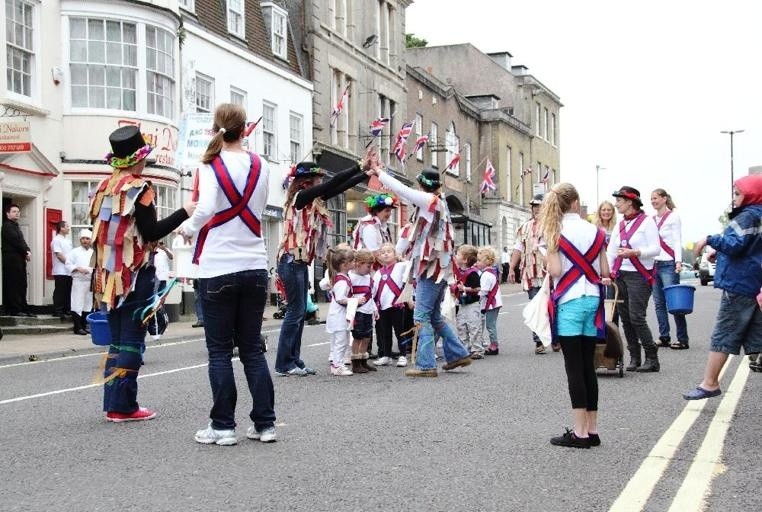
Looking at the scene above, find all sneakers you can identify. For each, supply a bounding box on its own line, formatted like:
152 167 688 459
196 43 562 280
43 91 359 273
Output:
195 421 238 445
551 341 562 352
749 354 762 372
107 406 156 423
330 346 498 376
550 426 601 449
536 342 545 354
275 366 317 376
246 421 277 442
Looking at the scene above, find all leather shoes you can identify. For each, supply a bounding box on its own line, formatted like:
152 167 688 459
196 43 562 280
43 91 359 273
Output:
14 311 38 317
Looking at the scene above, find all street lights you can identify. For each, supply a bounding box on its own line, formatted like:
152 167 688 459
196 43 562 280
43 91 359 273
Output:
595 163 606 209
720 129 747 216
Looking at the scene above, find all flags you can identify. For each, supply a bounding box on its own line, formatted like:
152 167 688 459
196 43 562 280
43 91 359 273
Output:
328 87 348 119
369 114 496 201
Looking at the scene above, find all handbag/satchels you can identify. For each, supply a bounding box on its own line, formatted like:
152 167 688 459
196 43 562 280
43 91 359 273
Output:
148 304 169 341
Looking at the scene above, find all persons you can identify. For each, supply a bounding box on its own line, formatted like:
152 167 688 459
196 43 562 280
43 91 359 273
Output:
174 103 278 445
682 172 762 400
50 220 207 340
272 192 503 376
2 204 38 317
532 181 612 447
501 180 689 372
87 126 197 423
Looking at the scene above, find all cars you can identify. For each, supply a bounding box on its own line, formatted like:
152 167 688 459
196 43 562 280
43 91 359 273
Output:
681 262 694 271
699 244 718 287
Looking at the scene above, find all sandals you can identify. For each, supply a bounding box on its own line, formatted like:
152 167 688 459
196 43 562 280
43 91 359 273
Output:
654 337 689 349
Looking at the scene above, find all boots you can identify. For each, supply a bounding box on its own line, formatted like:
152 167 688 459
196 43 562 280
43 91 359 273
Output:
70 310 91 334
626 343 641 371
637 342 660 372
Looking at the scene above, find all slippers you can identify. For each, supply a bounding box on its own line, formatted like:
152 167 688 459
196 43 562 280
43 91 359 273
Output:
682 386 722 400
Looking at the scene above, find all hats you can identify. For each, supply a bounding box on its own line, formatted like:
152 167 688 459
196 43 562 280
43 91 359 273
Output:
291 162 334 178
611 186 644 206
370 193 398 211
416 168 443 189
107 125 156 170
529 192 544 205
78 229 92 239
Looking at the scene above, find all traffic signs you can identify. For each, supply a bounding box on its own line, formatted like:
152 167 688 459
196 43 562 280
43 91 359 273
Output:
0 121 31 152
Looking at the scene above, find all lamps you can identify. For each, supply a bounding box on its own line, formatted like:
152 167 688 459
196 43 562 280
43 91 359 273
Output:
364 35 378 49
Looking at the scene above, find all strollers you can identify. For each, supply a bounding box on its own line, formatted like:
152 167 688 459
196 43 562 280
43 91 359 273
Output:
270 266 318 320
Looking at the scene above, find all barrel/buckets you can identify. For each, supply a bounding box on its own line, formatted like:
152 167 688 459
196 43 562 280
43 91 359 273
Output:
662 269 695 314
85 292 113 346
